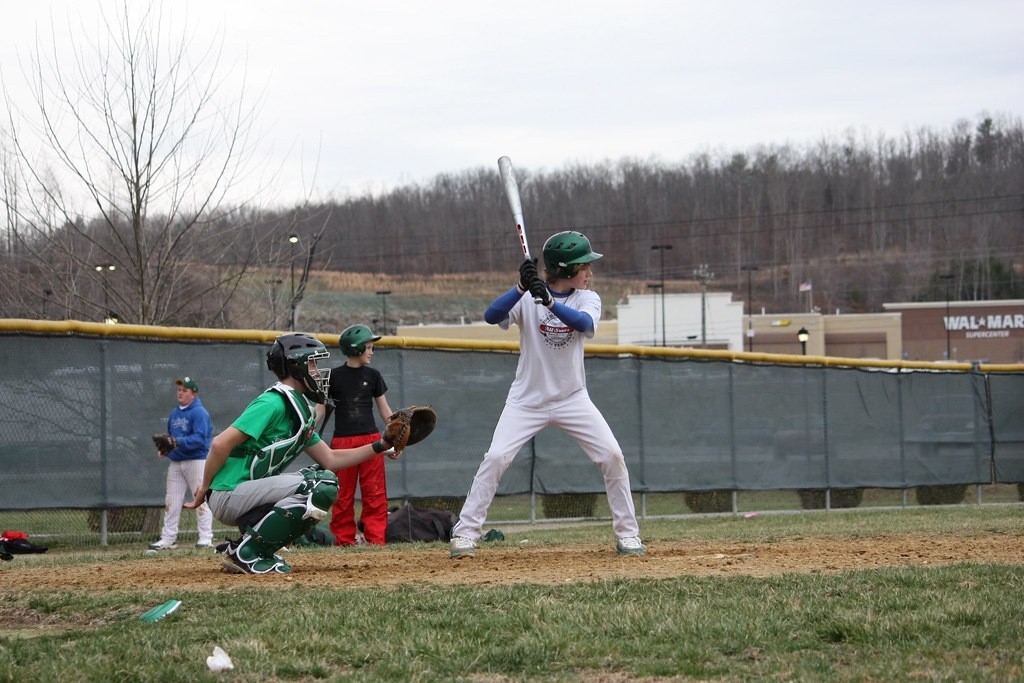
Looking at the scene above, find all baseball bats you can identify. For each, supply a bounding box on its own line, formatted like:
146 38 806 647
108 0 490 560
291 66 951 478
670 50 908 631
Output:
498 155 544 305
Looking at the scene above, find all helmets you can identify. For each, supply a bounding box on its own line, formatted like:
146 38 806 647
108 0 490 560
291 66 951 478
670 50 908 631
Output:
542 231 604 274
266 333 331 404
339 325 382 357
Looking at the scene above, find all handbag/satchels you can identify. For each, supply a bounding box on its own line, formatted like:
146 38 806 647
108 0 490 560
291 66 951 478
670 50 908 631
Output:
386 501 459 542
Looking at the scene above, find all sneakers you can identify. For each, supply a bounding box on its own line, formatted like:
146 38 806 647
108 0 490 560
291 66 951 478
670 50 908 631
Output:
148 539 177 550
194 537 212 549
215 541 255 575
616 535 645 556
449 536 476 558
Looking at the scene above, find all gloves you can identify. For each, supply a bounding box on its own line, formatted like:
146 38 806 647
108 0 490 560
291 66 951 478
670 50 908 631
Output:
528 276 552 306
519 258 539 291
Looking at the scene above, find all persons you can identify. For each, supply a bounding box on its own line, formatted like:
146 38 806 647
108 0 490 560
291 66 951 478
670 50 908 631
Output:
148 376 215 551
450 231 646 559
183 332 409 574
313 324 404 545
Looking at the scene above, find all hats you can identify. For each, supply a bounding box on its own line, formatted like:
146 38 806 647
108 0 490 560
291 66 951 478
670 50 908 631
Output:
176 376 199 393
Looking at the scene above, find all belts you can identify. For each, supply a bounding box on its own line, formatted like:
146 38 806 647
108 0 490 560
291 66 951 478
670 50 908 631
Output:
206 489 212 502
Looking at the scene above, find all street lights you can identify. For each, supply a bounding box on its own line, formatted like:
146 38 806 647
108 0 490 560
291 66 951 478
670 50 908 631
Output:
95 263 116 315
377 291 391 336
648 285 663 347
740 266 759 352
289 234 298 332
797 326 809 355
696 276 706 349
652 245 672 348
264 280 282 331
939 275 955 360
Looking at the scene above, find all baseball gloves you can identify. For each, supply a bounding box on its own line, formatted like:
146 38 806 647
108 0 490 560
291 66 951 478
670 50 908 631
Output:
152 432 177 457
380 404 438 460
